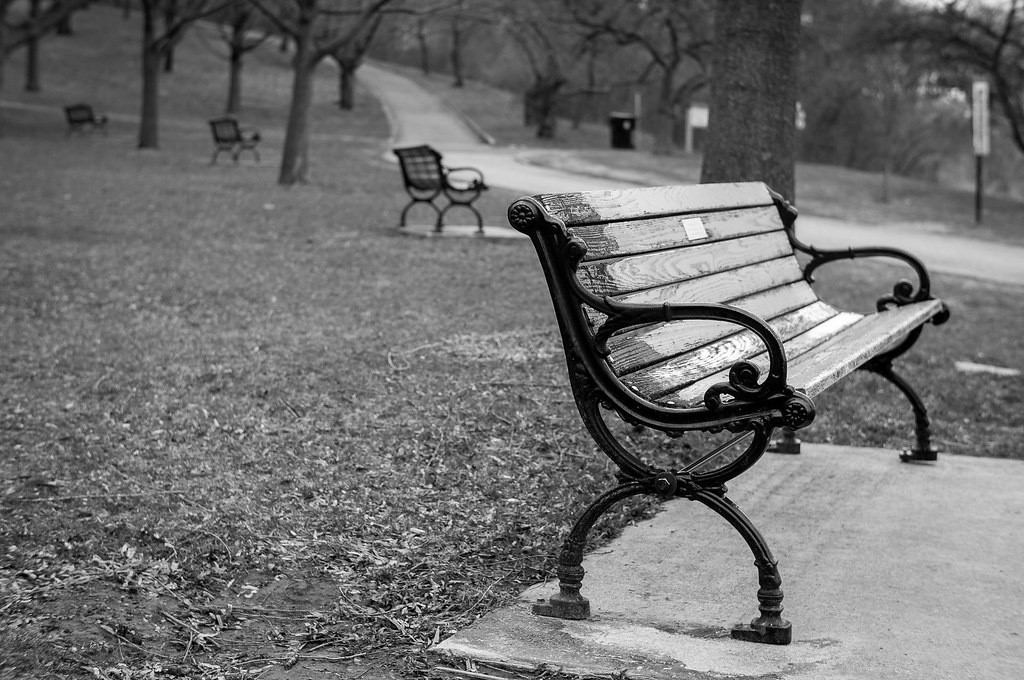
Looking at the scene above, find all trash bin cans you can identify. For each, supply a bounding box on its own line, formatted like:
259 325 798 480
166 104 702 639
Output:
609 109 636 151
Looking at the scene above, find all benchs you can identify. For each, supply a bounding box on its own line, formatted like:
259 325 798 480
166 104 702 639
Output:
207 116 262 166
393 143 487 236
507 180 951 643
64 102 110 135
